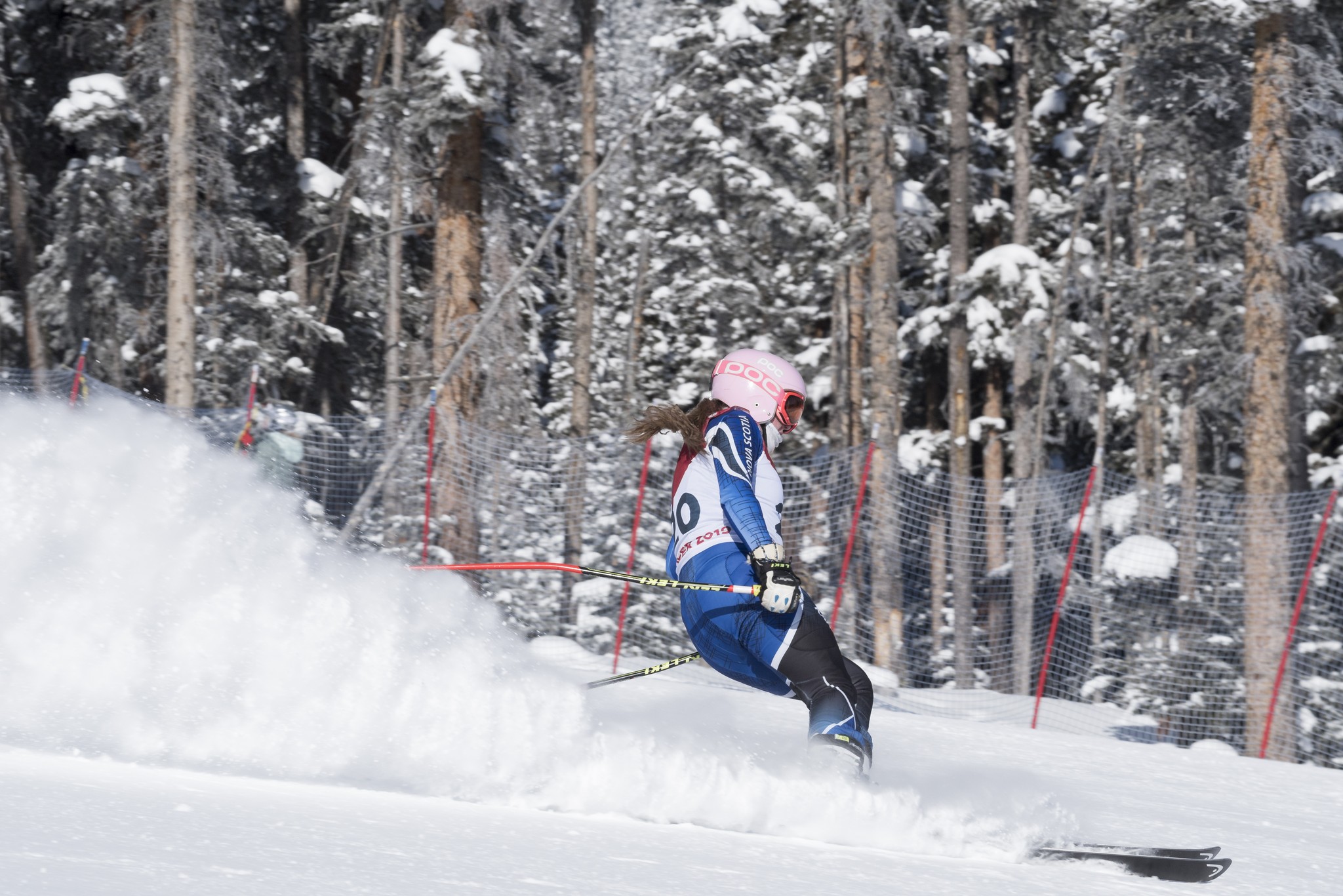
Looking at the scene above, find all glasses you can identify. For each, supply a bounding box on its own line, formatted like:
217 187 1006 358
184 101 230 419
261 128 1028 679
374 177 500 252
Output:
778 392 806 434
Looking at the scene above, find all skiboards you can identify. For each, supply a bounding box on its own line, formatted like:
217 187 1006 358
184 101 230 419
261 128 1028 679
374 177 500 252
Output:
1011 841 1232 883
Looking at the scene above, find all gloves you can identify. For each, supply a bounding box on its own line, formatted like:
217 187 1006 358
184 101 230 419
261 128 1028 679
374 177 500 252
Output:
751 545 802 614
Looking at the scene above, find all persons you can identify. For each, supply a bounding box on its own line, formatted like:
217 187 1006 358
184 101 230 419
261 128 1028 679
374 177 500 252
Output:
623 348 874 778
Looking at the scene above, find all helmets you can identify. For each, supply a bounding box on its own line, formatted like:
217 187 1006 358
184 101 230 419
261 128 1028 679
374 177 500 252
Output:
711 348 808 424
